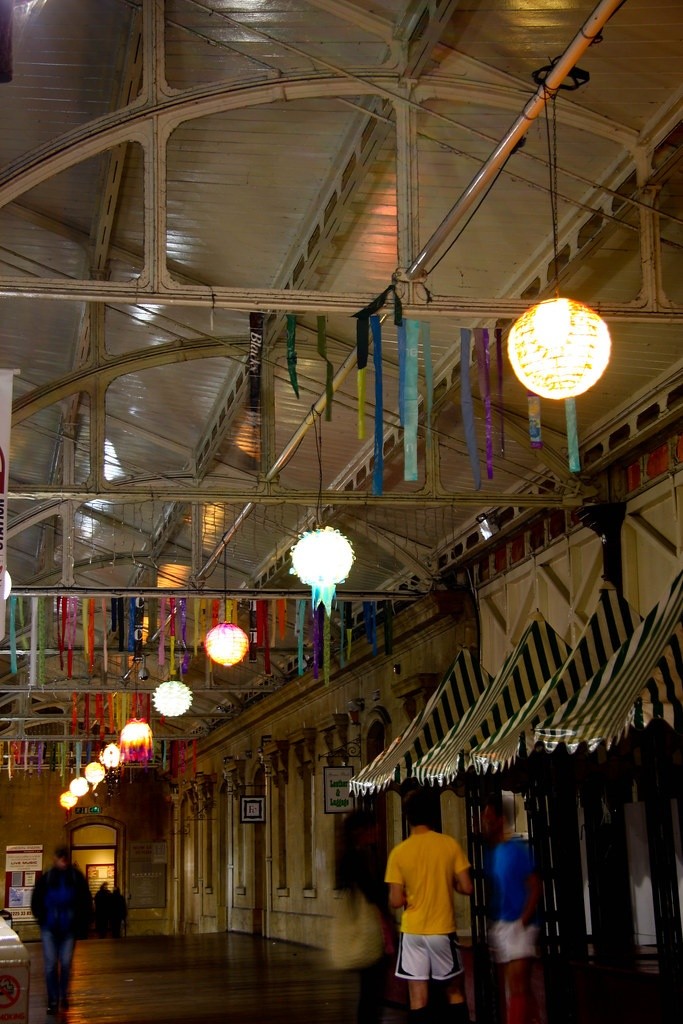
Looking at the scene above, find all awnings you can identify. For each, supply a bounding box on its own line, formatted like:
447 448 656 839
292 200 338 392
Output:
411 605 571 786
347 645 494 798
463 579 644 775
531 564 682 752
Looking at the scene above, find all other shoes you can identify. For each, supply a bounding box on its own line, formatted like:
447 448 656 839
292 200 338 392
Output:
45 1002 60 1017
59 992 70 1011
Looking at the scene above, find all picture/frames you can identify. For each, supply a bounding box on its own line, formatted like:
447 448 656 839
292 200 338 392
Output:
323 766 356 814
240 794 266 824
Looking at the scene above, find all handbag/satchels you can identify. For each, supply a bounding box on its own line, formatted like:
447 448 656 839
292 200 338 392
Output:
329 893 383 970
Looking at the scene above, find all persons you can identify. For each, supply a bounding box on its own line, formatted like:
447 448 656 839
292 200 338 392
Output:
383 790 475 1024
30 845 94 1015
93 880 127 938
482 794 547 1024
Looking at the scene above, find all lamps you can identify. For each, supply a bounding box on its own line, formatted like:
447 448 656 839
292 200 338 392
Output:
204 545 249 667
151 681 194 718
507 85 611 400
59 722 155 813
289 415 360 589
